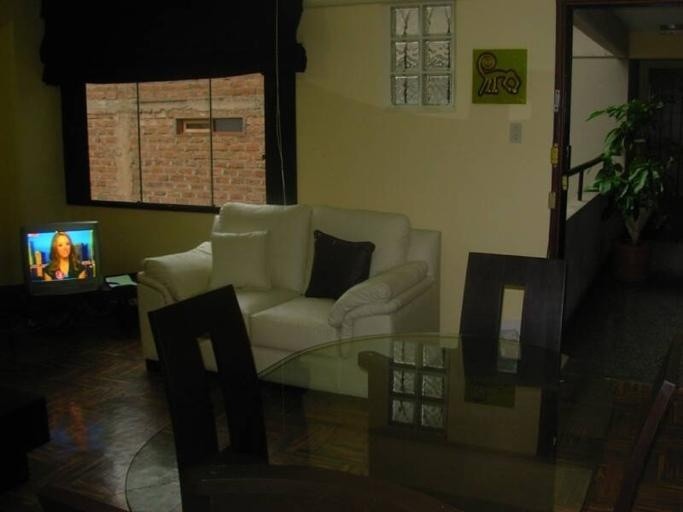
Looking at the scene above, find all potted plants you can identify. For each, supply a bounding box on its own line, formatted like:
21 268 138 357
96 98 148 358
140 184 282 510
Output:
582 84 683 288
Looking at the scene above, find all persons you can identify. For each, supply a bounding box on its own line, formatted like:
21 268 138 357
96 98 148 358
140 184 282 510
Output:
42 233 87 282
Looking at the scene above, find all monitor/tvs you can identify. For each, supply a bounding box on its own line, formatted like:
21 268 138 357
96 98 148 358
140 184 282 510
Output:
20 220 104 297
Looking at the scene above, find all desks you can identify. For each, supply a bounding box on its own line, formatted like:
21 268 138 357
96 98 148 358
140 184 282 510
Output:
125 332 614 512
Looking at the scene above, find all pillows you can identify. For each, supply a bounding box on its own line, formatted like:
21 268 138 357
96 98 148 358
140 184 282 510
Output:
304 228 375 299
210 229 269 286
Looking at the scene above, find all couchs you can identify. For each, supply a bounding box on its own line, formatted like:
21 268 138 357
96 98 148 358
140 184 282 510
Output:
136 202 441 399
